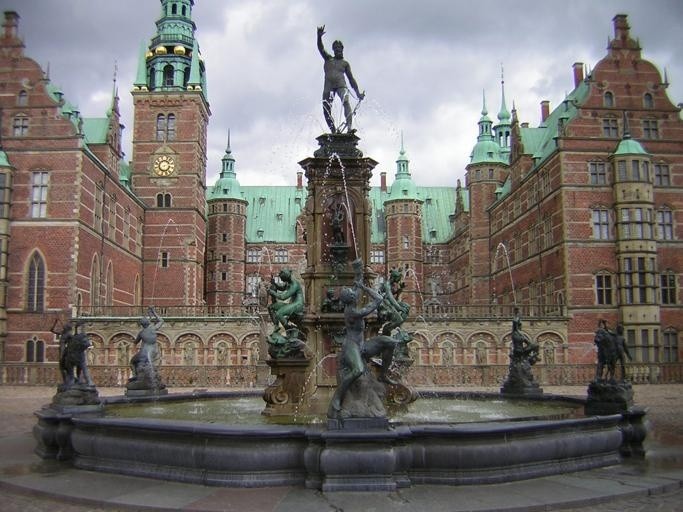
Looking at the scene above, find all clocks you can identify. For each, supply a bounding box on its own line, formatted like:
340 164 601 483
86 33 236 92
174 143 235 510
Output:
152 155 175 178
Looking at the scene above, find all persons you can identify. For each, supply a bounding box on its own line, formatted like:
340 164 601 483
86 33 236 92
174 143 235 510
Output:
126 304 162 382
314 24 365 135
49 317 72 380
375 262 407 335
329 256 398 413
263 269 304 336
599 318 631 380
507 305 542 363
